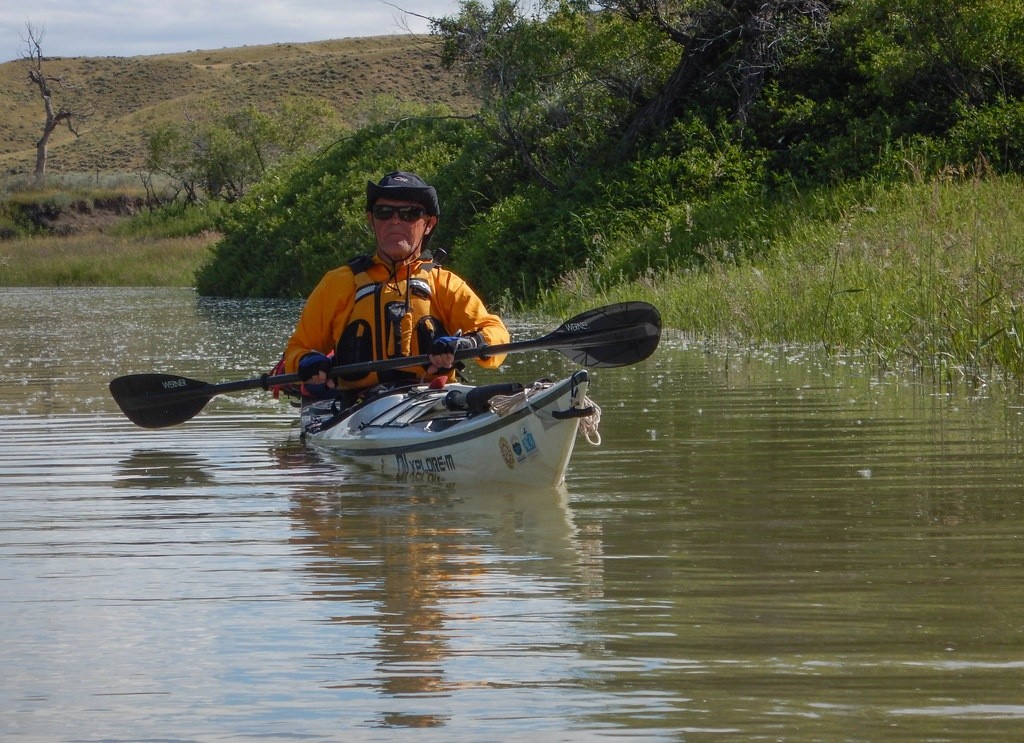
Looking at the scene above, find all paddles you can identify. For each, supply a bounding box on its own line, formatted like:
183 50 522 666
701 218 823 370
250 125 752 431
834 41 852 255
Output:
103 298 662 426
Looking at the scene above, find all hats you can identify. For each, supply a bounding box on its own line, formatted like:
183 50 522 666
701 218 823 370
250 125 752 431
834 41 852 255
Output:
365 172 440 215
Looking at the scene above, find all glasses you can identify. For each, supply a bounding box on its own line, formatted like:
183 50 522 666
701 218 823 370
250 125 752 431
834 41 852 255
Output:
373 204 424 223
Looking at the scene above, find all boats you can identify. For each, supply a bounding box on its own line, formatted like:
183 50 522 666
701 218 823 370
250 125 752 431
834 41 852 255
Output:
296 367 599 501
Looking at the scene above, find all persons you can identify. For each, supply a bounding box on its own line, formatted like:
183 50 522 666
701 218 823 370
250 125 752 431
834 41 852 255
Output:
283 171 510 416
